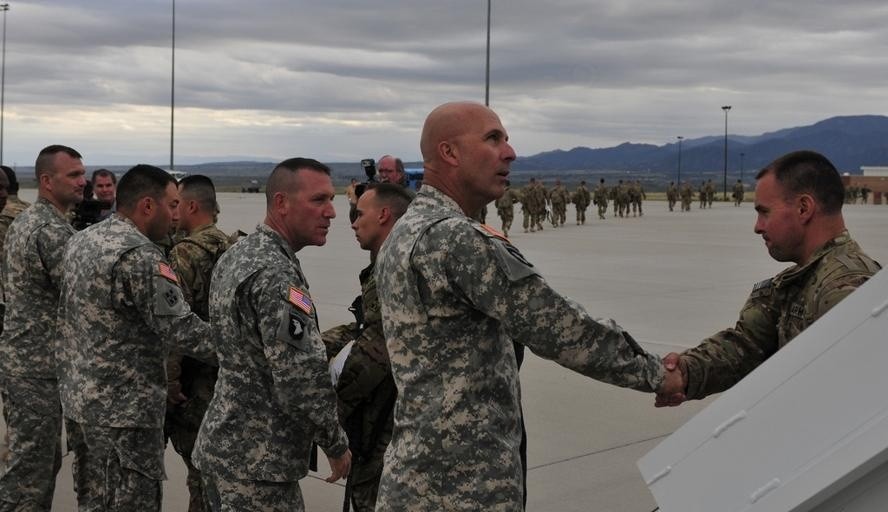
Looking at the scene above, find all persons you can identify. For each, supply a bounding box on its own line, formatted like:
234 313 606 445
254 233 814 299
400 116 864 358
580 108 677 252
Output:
337 183 416 512
699 179 707 209
594 177 608 219
347 179 359 224
477 206 488 225
1 145 87 512
861 183 873 204
91 169 117 223
654 150 882 408
190 157 352 512
732 178 744 206
520 176 540 233
707 179 715 208
0 166 31 249
852 182 860 205
666 181 677 211
415 181 422 192
495 179 519 237
572 181 590 225
626 180 633 215
535 181 550 231
550 179 571 228
167 174 248 512
54 165 219 511
846 186 852 205
0 167 10 213
373 102 684 511
611 180 626 217
633 180 646 217
69 180 97 231
679 180 691 211
378 155 406 188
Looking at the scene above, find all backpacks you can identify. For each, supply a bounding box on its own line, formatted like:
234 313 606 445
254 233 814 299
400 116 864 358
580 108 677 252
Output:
551 188 561 203
498 187 511 209
570 190 580 204
183 228 248 390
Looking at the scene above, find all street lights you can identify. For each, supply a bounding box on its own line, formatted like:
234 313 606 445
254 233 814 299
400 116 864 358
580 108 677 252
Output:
675 104 744 201
0 2 10 166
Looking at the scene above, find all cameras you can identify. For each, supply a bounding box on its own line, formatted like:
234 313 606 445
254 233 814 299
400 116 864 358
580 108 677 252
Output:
354 157 389 198
73 180 112 228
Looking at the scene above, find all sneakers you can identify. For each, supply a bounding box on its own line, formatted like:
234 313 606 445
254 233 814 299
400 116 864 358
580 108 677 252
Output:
503 227 508 237
524 228 528 233
530 227 534 231
